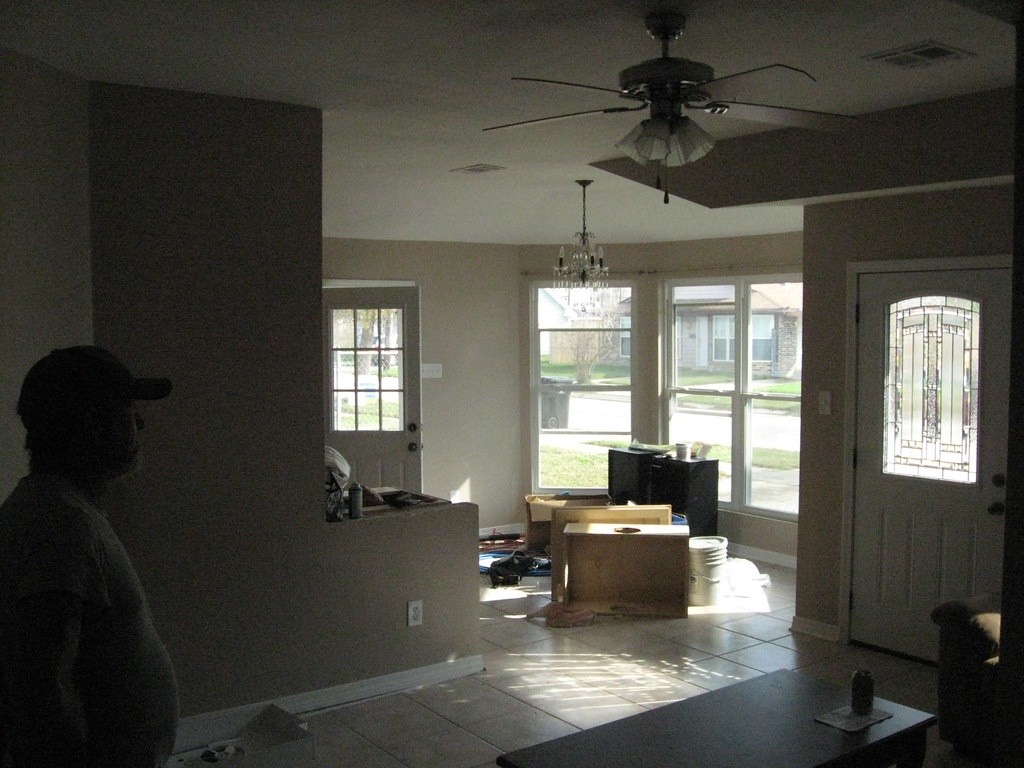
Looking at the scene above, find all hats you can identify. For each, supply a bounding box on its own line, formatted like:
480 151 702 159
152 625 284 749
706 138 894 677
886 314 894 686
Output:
15 345 173 418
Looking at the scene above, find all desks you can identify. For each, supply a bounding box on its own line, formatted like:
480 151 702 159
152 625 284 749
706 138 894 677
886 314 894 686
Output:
495 668 938 768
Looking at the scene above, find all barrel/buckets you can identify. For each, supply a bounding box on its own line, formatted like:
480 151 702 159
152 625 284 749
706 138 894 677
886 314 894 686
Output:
688 535 728 605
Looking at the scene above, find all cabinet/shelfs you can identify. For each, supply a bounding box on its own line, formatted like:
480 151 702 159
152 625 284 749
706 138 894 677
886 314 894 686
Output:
607 449 718 538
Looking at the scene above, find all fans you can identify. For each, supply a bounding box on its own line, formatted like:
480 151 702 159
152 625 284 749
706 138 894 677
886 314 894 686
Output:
482 10 859 136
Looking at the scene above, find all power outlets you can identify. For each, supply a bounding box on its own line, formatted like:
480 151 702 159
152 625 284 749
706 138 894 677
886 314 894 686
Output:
408 600 423 627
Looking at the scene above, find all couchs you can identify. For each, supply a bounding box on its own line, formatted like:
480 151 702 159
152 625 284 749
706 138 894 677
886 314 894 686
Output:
930 587 1003 752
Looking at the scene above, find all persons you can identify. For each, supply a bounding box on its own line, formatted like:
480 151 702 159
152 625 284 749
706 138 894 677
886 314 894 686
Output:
0 345 179 768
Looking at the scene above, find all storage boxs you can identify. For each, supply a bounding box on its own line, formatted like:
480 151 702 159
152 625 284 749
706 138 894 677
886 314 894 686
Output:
552 503 691 620
525 495 613 550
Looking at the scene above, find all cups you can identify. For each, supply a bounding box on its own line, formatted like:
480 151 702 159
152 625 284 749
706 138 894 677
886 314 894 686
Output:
676 443 688 460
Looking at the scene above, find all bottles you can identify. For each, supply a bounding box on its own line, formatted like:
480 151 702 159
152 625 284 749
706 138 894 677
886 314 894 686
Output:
349 481 363 519
852 669 874 714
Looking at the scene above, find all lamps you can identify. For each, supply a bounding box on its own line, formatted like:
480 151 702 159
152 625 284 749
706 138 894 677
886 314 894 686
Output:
551 180 610 304
616 114 715 204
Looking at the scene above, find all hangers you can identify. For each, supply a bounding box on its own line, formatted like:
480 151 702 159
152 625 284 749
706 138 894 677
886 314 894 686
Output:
480 529 525 551
